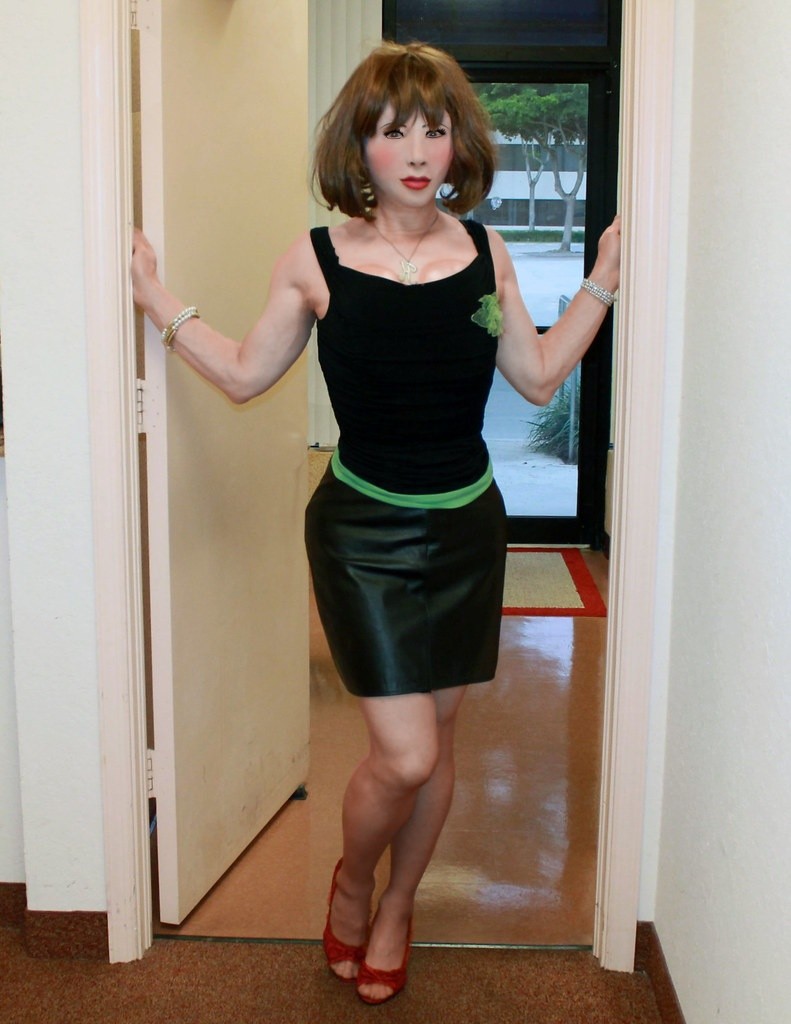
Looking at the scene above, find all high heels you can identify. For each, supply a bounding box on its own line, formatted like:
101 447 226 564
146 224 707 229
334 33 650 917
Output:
356 894 411 1005
323 857 368 978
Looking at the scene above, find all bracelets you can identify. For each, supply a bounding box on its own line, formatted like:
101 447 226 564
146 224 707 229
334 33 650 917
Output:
581 276 616 308
161 306 201 353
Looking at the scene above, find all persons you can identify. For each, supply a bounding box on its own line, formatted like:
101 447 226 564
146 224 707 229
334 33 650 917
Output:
132 42 621 1007
369 207 439 284
